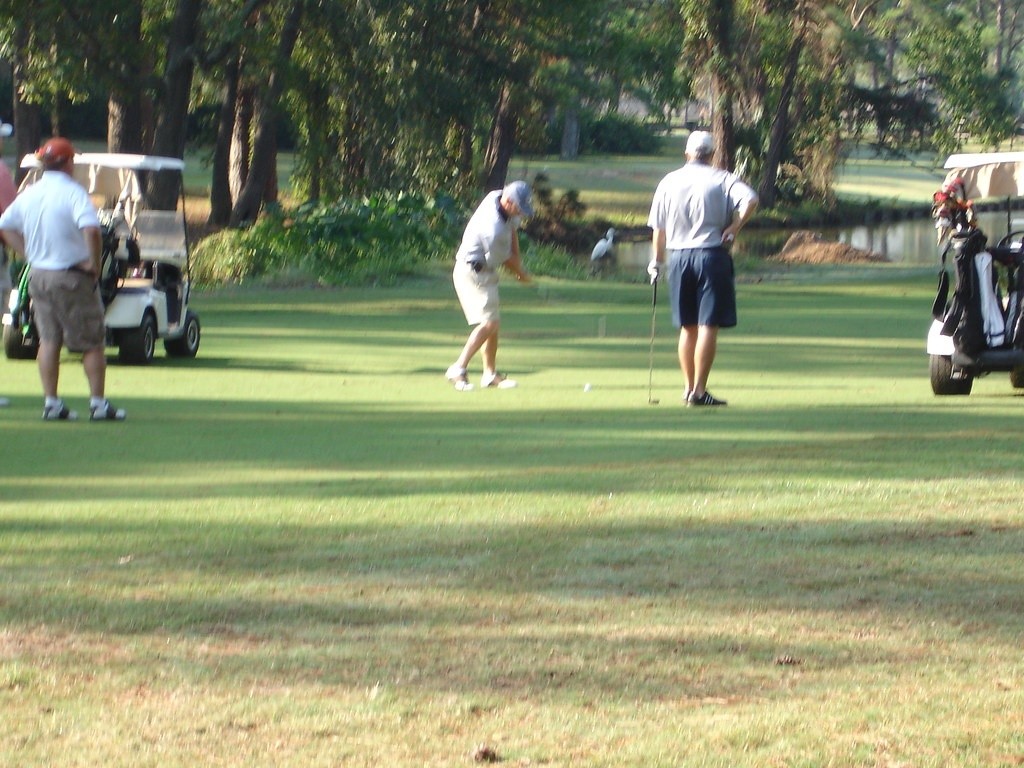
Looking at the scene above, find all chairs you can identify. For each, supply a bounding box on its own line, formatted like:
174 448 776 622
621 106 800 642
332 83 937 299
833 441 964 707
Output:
113 209 188 273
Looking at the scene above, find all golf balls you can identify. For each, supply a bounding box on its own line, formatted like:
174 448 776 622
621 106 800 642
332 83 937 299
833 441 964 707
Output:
584 383 591 393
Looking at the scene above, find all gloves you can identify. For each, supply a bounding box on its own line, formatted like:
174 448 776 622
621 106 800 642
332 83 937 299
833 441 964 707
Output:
648 260 663 285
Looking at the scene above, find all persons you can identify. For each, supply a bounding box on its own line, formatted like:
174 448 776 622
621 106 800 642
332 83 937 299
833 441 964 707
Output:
646 130 759 405
0 137 126 422
445 180 534 391
0 122 19 408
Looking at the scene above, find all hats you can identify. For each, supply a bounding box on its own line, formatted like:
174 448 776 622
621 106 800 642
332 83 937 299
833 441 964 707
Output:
505 181 534 217
687 130 716 154
40 138 82 163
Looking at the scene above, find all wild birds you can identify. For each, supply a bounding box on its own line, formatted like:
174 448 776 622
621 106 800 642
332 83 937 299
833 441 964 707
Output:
591 228 620 262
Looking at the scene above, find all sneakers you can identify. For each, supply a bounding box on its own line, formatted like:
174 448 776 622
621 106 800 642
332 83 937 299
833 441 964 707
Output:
0 397 11 406
88 401 126 420
445 365 474 390
43 400 78 420
684 389 726 406
481 373 516 388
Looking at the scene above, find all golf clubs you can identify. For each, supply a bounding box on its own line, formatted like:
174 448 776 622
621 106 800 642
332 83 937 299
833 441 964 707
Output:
96 203 125 238
647 267 661 406
930 177 977 247
